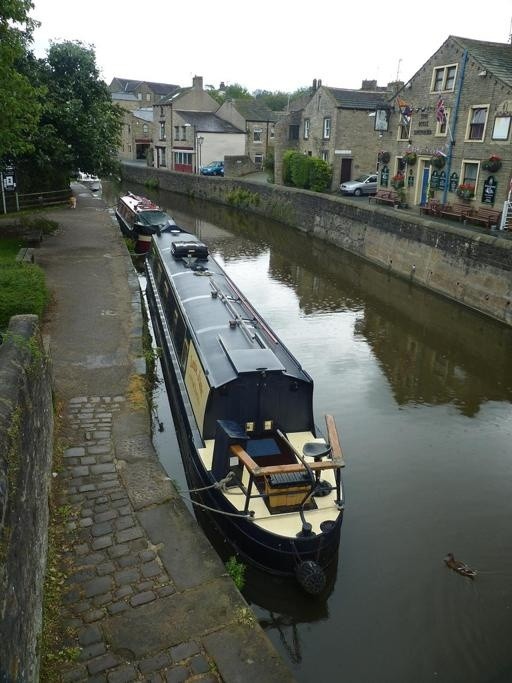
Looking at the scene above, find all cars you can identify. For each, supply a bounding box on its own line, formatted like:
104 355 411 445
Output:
340 174 378 197
201 160 225 175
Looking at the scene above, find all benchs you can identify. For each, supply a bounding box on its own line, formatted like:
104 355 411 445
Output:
16 247 37 264
367 188 403 206
420 195 502 229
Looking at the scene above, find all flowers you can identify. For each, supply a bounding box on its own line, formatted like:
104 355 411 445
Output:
390 175 404 189
457 182 475 194
481 153 503 170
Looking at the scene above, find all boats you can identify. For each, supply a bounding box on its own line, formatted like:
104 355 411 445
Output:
143 220 344 593
77 171 102 190
117 191 180 252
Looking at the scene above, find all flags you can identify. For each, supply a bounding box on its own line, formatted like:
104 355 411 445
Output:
436 94 445 123
397 97 411 126
